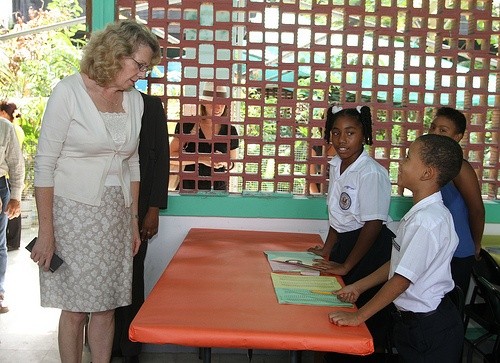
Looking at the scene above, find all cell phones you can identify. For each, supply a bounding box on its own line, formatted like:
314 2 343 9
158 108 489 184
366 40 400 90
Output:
26 237 63 273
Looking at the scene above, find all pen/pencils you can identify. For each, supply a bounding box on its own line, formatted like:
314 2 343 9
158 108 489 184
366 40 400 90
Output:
311 290 332 295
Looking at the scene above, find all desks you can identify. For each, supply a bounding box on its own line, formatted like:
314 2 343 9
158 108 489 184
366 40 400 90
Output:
128 227 374 363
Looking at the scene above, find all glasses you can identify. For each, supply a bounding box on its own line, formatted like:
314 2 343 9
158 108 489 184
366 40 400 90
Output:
127 54 152 73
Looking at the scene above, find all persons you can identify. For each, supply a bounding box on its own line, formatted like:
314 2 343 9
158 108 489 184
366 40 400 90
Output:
104 86 170 362
428 107 485 319
307 105 395 363
306 111 332 196
327 133 464 363
168 79 239 192
0 101 25 313
31 18 162 363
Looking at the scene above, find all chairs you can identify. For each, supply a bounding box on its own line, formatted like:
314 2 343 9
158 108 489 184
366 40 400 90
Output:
465 245 500 363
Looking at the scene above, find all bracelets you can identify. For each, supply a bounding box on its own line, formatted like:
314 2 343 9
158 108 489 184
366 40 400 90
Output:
223 159 235 170
